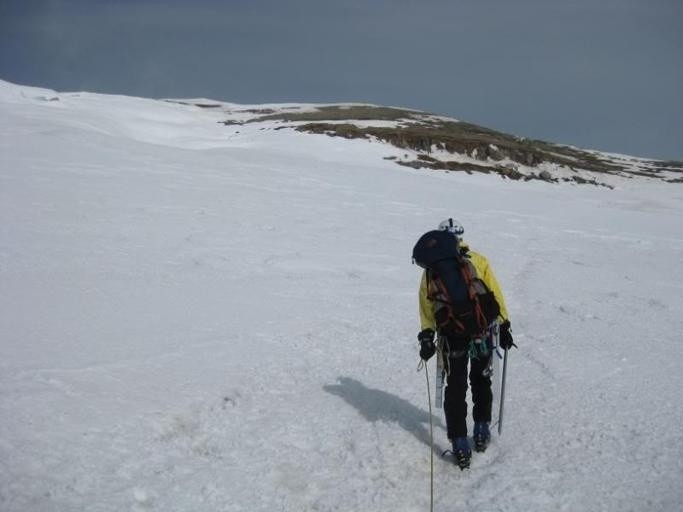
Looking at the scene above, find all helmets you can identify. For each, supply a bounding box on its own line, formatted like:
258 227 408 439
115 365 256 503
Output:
439 218 465 240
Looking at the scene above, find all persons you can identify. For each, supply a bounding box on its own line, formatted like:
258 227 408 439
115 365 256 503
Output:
417 218 513 470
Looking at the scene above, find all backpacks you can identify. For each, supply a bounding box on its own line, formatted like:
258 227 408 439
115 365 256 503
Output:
413 230 500 339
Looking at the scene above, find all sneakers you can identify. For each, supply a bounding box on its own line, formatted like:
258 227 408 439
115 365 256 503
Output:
452 439 472 463
473 423 487 446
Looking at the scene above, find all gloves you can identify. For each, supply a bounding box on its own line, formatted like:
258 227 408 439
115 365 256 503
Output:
418 328 436 360
499 321 513 350
